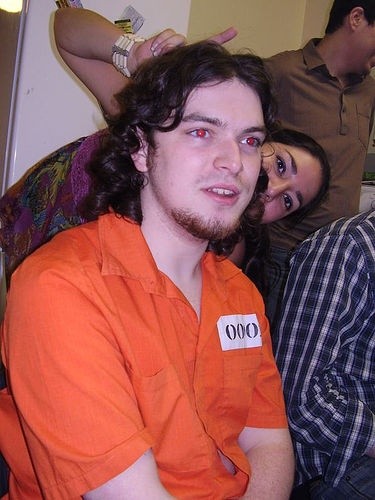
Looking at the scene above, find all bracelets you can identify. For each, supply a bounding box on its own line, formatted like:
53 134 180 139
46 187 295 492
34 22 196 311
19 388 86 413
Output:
112 32 146 78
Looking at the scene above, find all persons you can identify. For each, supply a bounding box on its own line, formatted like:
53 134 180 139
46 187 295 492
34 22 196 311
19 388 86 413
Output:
0 0 375 500
